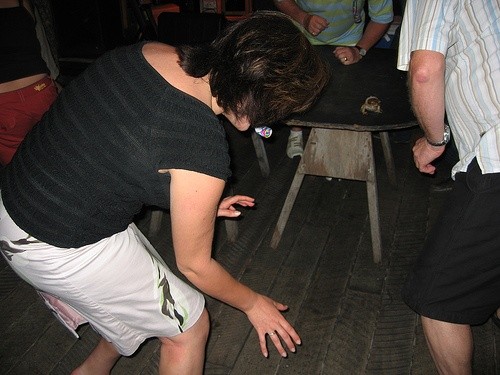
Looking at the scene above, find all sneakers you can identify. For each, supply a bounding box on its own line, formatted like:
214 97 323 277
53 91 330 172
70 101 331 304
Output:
285 133 304 161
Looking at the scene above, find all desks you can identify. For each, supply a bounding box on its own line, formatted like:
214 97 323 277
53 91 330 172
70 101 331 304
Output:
267 45 418 264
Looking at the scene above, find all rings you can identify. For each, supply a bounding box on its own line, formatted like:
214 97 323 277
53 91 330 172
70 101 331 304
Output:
269 332 275 336
344 58 347 61
311 32 315 36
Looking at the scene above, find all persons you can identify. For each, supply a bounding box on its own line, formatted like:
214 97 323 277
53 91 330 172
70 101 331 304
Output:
274 0 394 162
397 0 500 374
0 18 329 375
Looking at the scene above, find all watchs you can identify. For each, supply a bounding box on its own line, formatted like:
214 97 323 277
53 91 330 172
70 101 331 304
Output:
354 45 367 57
425 125 451 146
